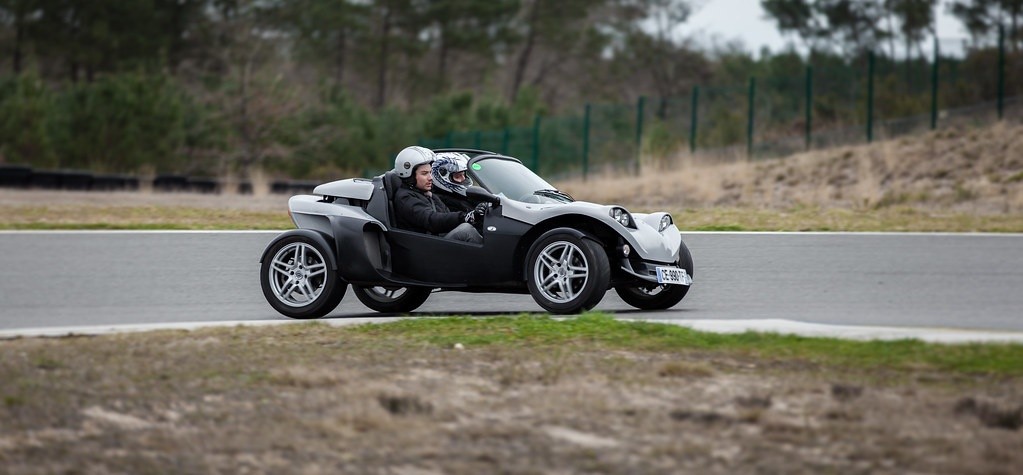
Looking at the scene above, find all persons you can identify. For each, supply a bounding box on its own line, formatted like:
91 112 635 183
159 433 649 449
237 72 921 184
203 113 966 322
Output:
392 146 490 243
431 152 480 212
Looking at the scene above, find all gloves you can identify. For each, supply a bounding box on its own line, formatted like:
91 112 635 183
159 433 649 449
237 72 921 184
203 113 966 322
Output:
464 202 487 225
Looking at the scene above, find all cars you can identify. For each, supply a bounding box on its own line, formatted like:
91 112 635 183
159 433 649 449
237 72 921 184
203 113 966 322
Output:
260 148 696 322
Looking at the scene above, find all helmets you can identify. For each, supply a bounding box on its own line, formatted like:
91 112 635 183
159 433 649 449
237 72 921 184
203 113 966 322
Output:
394 146 436 178
432 152 473 197
